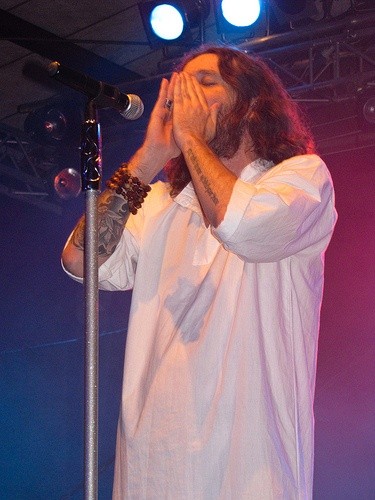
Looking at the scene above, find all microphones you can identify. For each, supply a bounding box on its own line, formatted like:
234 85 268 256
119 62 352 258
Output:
48 62 144 120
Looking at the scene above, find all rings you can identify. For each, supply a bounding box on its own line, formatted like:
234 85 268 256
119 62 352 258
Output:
165 98 172 107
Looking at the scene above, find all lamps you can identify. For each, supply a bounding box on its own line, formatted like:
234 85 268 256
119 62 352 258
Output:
23 102 72 142
49 164 83 203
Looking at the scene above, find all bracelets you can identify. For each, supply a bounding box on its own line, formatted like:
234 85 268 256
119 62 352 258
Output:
105 161 152 215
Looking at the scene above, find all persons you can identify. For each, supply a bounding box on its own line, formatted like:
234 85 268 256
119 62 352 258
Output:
60 46 339 500
